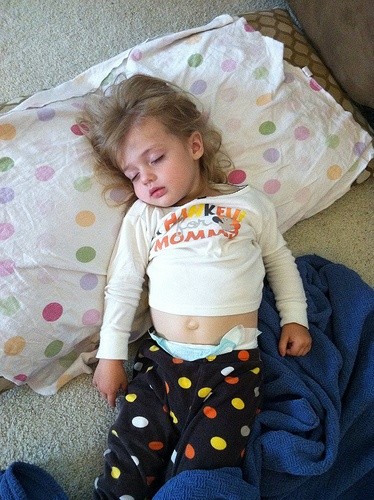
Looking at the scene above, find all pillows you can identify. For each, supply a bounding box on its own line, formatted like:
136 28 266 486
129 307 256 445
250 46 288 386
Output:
0 8 373 398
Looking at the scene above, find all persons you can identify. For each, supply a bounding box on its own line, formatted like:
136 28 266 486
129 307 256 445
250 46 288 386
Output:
76 75 313 500
